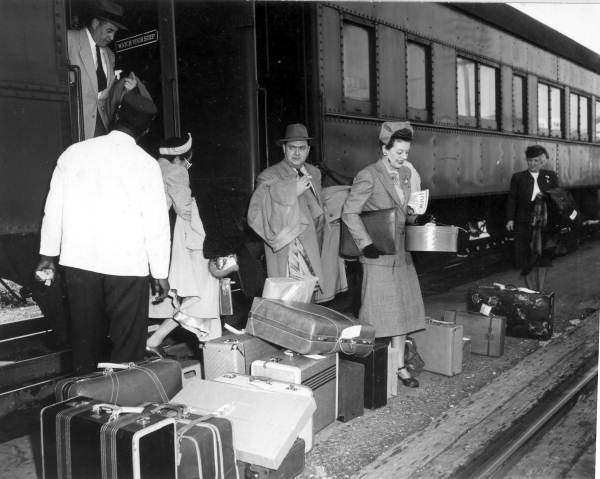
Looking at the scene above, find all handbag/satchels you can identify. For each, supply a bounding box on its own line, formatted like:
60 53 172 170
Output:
338 207 398 257
219 277 234 316
404 335 425 376
466 279 555 341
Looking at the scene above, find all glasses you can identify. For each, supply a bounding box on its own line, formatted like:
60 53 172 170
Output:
181 156 193 169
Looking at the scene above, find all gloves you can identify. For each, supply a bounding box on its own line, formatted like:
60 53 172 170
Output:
362 243 384 259
417 214 432 225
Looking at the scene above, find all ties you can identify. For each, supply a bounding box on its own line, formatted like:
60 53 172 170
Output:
296 167 316 197
95 44 107 92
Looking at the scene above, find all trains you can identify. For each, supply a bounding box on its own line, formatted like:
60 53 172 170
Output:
0 0 600 303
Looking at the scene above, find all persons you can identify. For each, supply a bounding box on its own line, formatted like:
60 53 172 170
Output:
342 121 430 387
254 123 323 278
34 94 171 375
67 0 137 142
506 146 558 291
144 138 193 360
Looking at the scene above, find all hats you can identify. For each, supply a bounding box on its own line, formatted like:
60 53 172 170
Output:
378 121 414 146
116 91 158 133
525 145 549 159
278 123 313 146
89 0 129 31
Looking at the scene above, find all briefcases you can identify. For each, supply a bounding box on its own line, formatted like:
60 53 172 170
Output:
337 339 388 410
455 310 508 357
40 394 178 479
386 347 399 400
250 348 339 437
407 316 463 377
336 358 366 423
134 401 240 479
55 356 182 406
245 297 376 359
212 371 313 455
202 328 280 381
167 375 318 471
236 438 305 479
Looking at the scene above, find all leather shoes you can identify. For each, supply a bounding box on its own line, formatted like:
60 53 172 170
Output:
144 345 177 360
398 366 419 388
